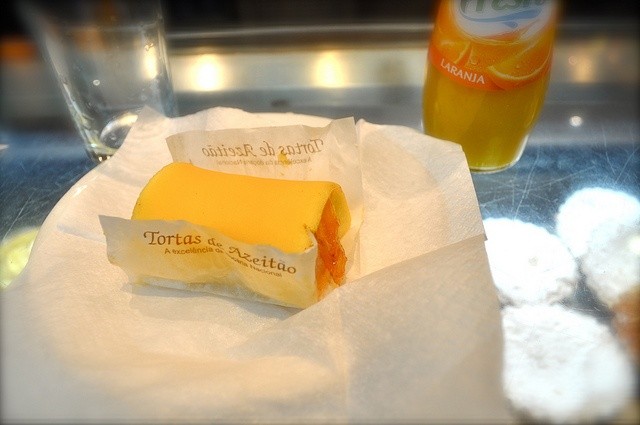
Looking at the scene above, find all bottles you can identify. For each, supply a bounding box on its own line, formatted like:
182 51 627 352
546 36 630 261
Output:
421 1 560 171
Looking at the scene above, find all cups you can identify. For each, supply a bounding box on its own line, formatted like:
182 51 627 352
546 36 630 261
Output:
21 1 179 163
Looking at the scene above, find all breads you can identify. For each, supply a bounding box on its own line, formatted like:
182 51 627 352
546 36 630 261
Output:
130 161 357 309
